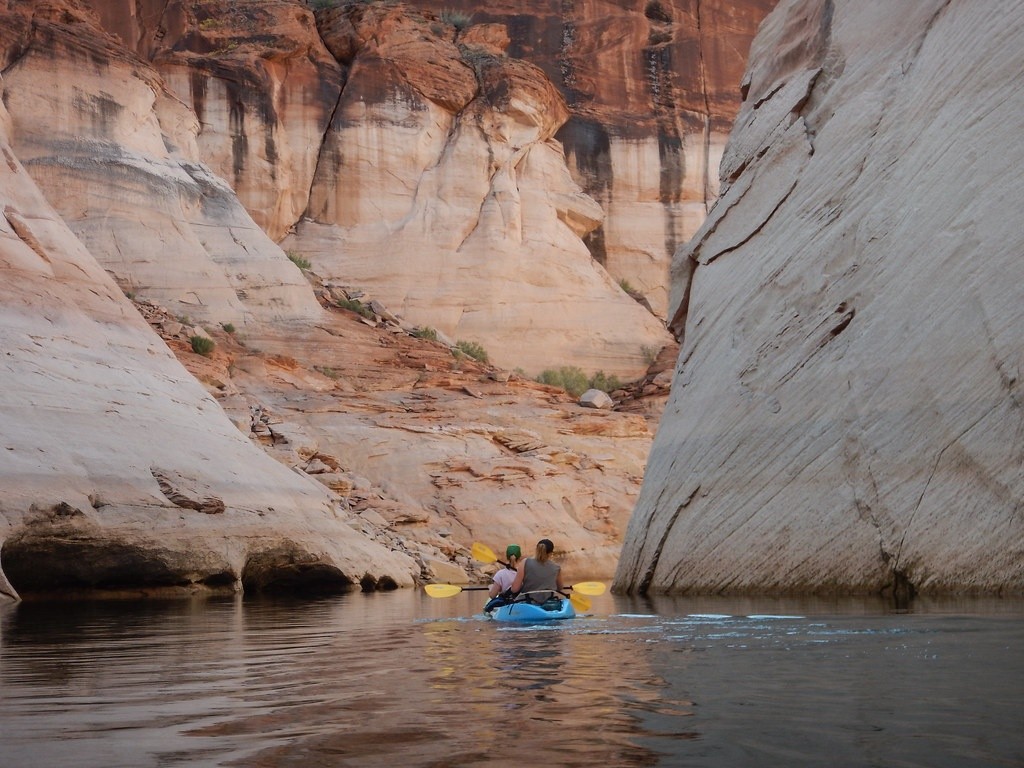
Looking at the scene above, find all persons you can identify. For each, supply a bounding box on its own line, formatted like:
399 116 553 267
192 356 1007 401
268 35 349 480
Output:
488 544 521 599
511 539 563 605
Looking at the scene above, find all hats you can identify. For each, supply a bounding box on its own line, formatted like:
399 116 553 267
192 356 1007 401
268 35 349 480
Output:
506 544 521 558
537 539 554 553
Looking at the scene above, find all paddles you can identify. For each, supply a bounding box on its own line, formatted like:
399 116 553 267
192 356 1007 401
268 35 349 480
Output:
423 580 607 599
469 541 592 613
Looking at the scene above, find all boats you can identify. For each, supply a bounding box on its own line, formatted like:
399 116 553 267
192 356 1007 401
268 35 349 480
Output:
494 597 577 622
